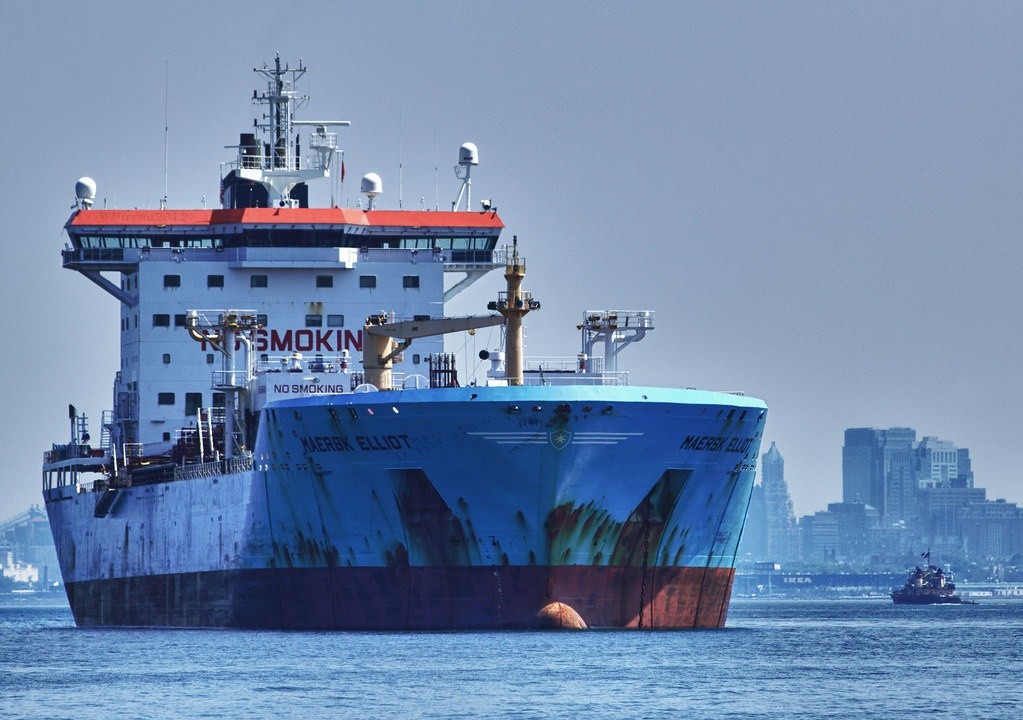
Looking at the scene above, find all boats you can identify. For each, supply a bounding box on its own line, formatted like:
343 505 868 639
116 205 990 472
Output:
889 547 964 605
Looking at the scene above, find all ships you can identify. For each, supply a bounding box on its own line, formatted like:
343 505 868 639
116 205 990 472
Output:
41 51 769 636
